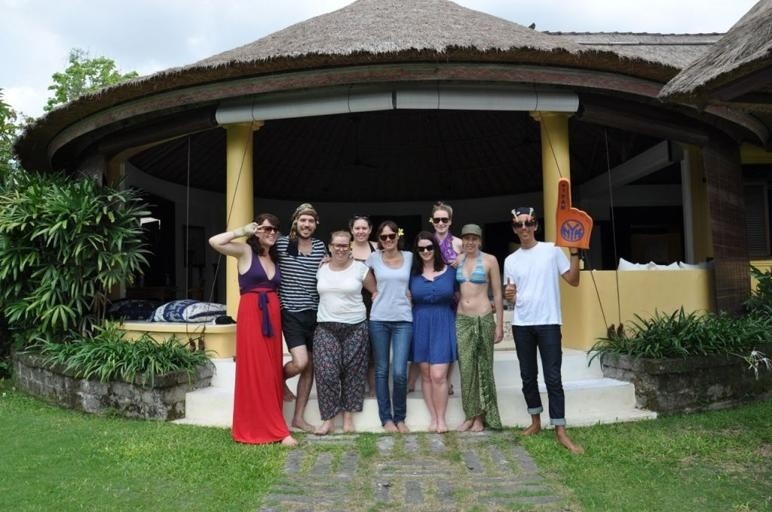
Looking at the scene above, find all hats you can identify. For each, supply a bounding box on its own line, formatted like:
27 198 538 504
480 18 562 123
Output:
510 206 536 219
459 224 482 238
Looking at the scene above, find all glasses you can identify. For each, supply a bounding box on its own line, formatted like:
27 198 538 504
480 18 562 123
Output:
380 232 396 241
432 217 450 223
352 212 370 220
512 219 536 228
330 243 350 251
417 244 433 252
258 226 279 234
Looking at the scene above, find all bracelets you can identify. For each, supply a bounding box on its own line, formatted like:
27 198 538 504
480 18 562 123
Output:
568 251 579 258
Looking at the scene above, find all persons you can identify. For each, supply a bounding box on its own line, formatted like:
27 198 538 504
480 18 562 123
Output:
410 230 459 436
341 210 383 399
407 199 466 395
319 220 459 434
276 203 329 434
311 231 376 436
500 204 585 456
454 222 505 432
207 212 300 445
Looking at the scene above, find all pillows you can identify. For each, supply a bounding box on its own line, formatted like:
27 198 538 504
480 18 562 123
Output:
617 257 715 271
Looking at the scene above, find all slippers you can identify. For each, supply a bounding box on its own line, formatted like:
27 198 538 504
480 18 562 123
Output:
407 385 414 392
448 384 453 395
